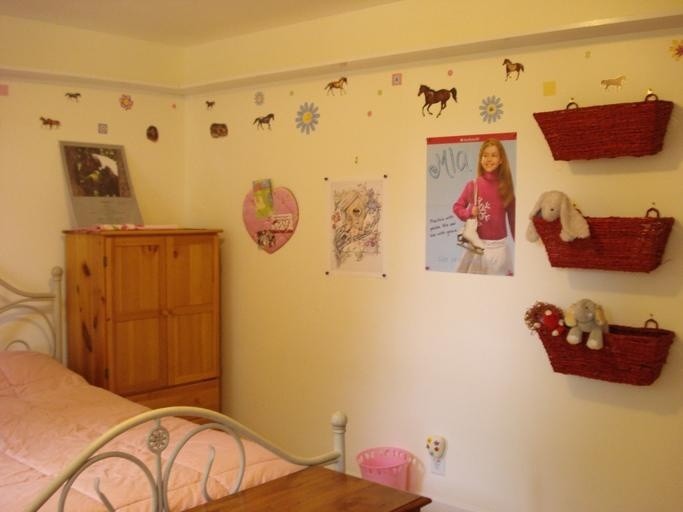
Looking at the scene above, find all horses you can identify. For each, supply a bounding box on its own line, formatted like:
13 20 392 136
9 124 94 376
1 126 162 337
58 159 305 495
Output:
253 113 274 129
502 58 524 81
205 101 215 111
601 75 627 96
39 116 59 130
324 77 348 96
65 92 81 103
418 85 457 117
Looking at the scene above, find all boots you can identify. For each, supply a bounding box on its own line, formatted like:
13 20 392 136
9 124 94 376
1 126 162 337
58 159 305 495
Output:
462 219 484 249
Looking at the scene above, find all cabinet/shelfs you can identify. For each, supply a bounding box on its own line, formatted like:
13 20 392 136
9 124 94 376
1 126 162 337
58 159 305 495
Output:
60 228 223 434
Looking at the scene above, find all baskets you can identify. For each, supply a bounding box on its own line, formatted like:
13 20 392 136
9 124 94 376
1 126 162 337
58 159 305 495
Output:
535 319 675 386
532 208 675 273
533 95 675 161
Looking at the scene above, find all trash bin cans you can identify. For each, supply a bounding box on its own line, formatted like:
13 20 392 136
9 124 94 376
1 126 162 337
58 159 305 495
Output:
356 447 412 491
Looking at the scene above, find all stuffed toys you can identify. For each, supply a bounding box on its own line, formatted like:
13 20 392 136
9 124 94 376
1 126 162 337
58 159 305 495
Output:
564 300 610 350
524 192 591 248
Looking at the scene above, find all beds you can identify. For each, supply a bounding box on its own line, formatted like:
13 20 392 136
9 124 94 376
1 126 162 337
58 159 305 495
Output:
0 266 347 511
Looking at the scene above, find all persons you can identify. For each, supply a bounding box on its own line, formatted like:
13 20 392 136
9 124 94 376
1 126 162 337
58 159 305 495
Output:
90 157 118 197
453 138 514 275
